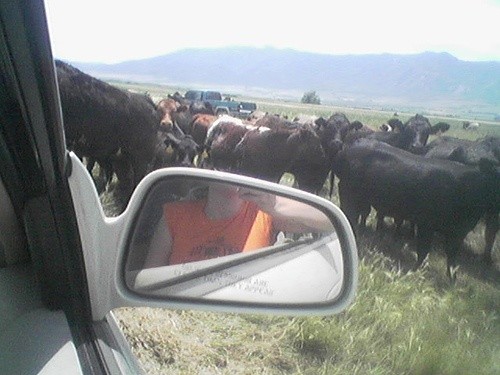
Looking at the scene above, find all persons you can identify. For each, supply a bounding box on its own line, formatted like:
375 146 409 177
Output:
143 181 334 267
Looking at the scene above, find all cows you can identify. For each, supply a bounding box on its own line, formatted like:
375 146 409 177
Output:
332 139 500 281
55 59 164 214
153 88 500 196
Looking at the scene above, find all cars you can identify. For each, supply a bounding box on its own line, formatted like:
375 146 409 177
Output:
1 0 359 374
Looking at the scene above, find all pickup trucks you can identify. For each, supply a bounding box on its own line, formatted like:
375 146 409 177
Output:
185 89 256 120
